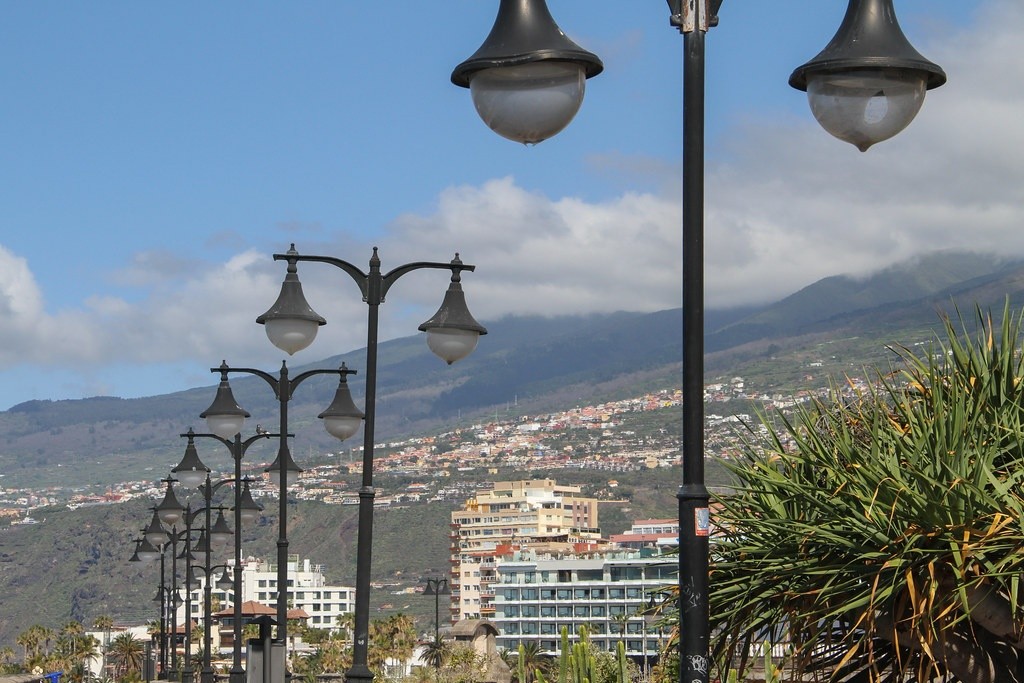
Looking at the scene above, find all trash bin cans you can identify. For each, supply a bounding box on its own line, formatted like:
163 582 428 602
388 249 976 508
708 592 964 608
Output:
246 615 286 683
45 672 62 683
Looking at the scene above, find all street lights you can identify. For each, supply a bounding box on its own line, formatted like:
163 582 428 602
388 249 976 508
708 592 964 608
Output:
198 360 366 683
129 472 263 683
422 576 451 673
255 240 488 683
450 0 948 683
171 427 303 683
